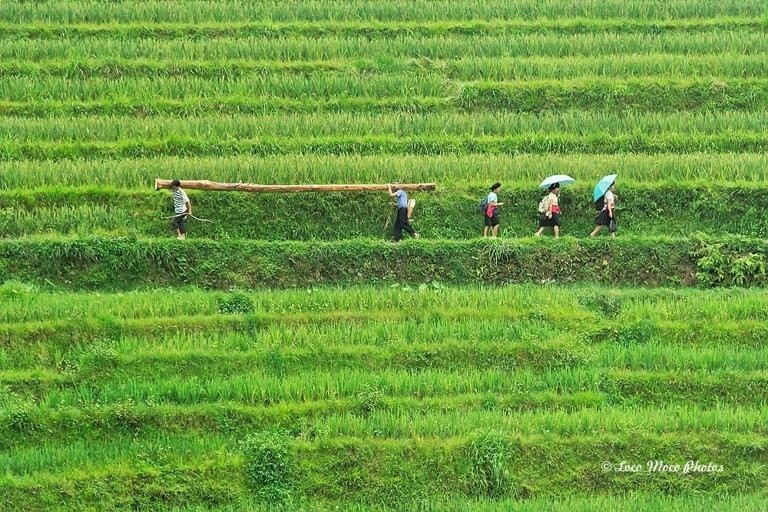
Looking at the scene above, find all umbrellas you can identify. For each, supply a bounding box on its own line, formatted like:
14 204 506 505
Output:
539 175 576 189
591 174 618 202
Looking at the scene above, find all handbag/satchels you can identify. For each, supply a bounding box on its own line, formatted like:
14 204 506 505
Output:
551 205 560 213
610 220 616 232
487 206 495 218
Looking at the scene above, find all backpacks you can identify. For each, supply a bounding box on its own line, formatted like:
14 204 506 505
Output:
480 197 487 211
595 195 604 211
539 196 549 213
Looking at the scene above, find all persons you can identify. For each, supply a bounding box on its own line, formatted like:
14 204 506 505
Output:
171 179 192 240
590 180 618 239
483 182 504 236
534 182 561 239
386 182 420 242
